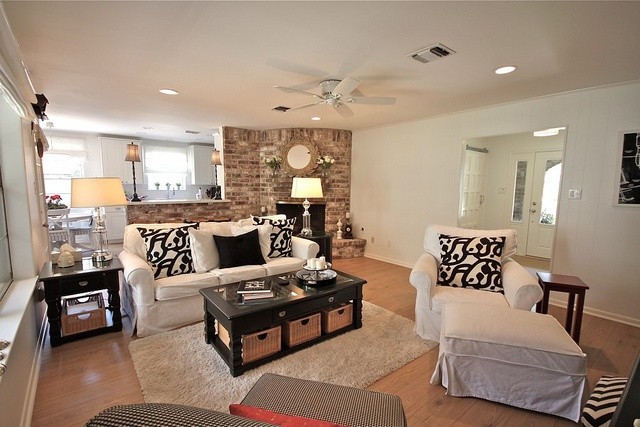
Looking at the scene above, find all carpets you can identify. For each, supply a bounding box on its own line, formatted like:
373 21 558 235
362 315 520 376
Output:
127 302 438 413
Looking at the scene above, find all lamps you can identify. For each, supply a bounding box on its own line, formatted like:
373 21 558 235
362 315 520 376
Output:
291 177 324 236
209 148 223 199
70 177 129 263
124 142 141 202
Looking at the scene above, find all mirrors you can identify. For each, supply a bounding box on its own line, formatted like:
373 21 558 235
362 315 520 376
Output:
279 135 321 178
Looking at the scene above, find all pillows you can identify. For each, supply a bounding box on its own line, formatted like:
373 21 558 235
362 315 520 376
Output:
225 404 344 426
249 214 297 258
213 228 267 269
187 227 220 272
136 224 200 278
432 230 505 295
231 225 273 261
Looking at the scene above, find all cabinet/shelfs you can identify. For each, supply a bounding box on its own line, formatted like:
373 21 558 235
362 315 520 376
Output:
47 209 70 246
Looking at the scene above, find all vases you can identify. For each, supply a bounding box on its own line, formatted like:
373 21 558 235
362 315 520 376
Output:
270 168 278 178
322 169 329 178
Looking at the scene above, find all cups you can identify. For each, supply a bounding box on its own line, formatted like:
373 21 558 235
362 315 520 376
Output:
316 260 325 269
310 258 319 268
307 260 310 267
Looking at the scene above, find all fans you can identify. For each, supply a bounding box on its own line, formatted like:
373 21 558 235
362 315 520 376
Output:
274 76 397 119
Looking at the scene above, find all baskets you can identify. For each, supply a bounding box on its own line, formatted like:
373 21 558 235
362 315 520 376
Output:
321 302 354 334
282 312 321 348
61 292 108 337
218 321 282 367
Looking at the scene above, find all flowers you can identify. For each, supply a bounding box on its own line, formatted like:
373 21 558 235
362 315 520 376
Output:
263 155 282 174
317 154 335 175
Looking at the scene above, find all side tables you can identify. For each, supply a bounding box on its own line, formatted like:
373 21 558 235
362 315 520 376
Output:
536 271 590 346
39 253 124 348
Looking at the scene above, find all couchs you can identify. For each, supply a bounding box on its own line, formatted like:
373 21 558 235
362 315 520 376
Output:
408 223 544 345
118 214 321 339
430 301 586 423
85 370 406 426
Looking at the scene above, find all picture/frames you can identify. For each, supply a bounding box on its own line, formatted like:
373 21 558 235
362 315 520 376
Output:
611 129 640 207
